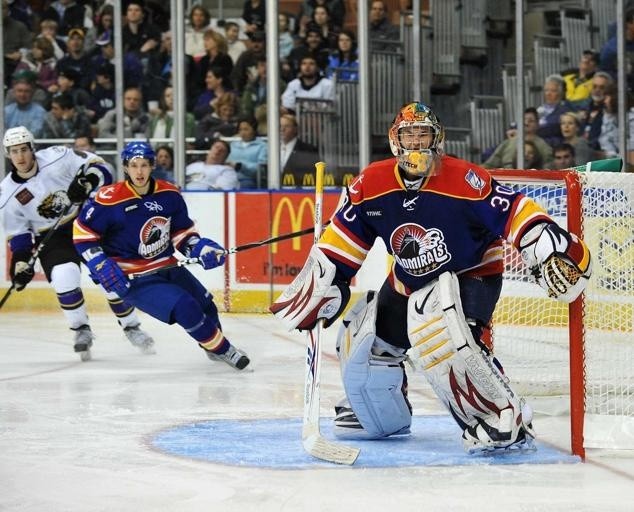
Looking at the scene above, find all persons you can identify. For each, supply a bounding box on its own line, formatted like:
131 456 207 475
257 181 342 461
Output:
71 142 250 372
269 103 591 456
0 125 149 352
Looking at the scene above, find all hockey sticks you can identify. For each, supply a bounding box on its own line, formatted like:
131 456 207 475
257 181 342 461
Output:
304 162 359 466
125 182 346 284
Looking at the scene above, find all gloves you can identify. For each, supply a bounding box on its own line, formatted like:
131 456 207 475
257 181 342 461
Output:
87 256 131 302
37 187 71 221
188 237 227 269
9 250 35 293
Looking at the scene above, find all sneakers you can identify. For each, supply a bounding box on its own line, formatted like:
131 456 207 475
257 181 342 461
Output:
73 328 94 353
122 326 154 350
203 343 250 370
485 413 533 450
333 399 411 434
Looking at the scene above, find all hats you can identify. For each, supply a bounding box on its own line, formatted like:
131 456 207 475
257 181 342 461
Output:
96 30 112 45
68 28 83 38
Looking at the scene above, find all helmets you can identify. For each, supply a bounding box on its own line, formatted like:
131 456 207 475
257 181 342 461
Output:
387 102 447 179
120 142 156 173
2 126 36 158
